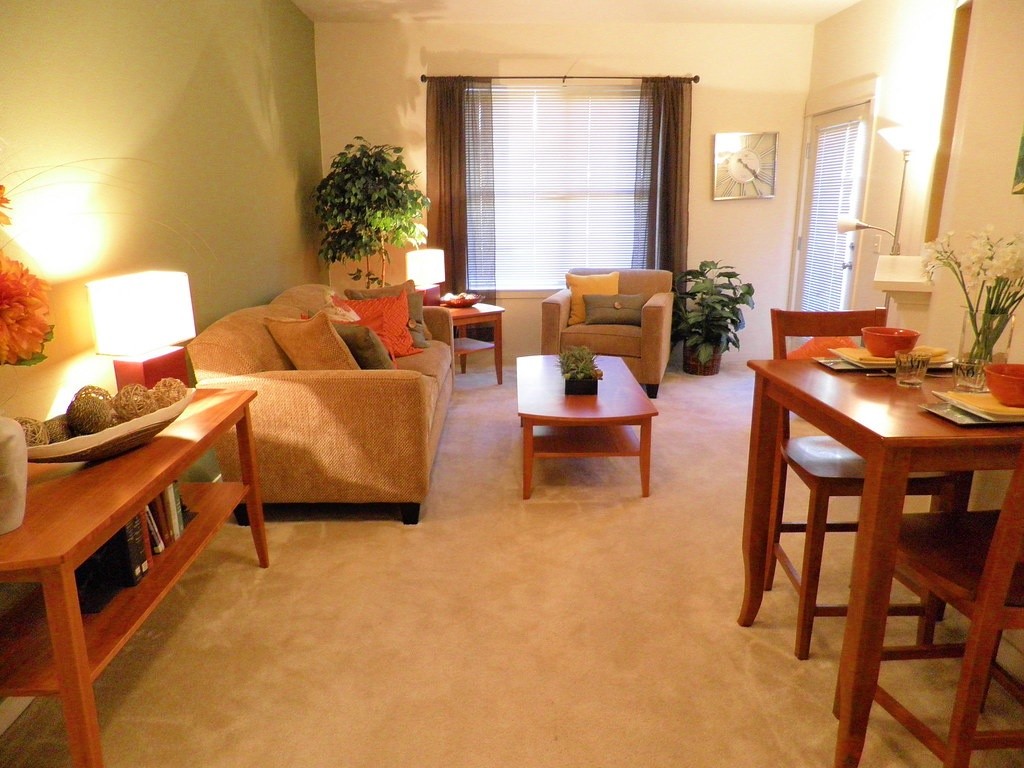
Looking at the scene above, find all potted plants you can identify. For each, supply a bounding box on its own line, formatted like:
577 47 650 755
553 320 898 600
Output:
672 260 755 376
554 345 604 396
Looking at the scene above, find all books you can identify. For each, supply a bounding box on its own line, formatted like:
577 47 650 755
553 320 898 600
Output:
111 480 186 587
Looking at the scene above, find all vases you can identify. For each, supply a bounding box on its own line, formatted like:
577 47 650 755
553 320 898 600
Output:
953 309 1016 396
0 410 28 536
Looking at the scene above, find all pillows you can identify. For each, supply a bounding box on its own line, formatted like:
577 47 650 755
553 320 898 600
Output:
565 272 645 326
263 280 432 370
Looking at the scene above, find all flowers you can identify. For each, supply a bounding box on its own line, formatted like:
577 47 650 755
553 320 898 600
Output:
918 225 1024 374
0 157 221 367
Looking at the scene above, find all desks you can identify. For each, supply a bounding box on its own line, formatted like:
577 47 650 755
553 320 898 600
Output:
0 389 269 767
738 359 1024 768
440 303 505 385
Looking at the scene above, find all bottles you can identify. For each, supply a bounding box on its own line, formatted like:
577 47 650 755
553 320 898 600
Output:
0 415 28 536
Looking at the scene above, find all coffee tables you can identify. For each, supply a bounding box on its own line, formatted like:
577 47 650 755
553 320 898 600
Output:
516 355 659 500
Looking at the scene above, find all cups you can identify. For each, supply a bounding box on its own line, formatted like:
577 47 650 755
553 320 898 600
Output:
895 350 931 391
952 360 986 393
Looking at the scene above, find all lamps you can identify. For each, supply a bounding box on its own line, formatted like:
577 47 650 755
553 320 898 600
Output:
87 270 196 393
405 249 445 306
837 125 932 307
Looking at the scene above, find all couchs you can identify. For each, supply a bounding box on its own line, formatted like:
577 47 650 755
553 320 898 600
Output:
187 283 455 524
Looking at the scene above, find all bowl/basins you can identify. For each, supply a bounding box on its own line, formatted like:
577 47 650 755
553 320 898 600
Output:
983 364 1024 407
861 327 921 358
440 294 486 308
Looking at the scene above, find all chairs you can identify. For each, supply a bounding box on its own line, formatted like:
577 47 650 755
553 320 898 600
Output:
542 267 675 399
766 307 1024 768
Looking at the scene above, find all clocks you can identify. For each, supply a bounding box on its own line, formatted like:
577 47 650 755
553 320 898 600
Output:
712 131 779 201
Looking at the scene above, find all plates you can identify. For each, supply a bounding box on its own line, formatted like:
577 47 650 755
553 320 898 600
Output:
932 390 1024 421
827 348 957 368
